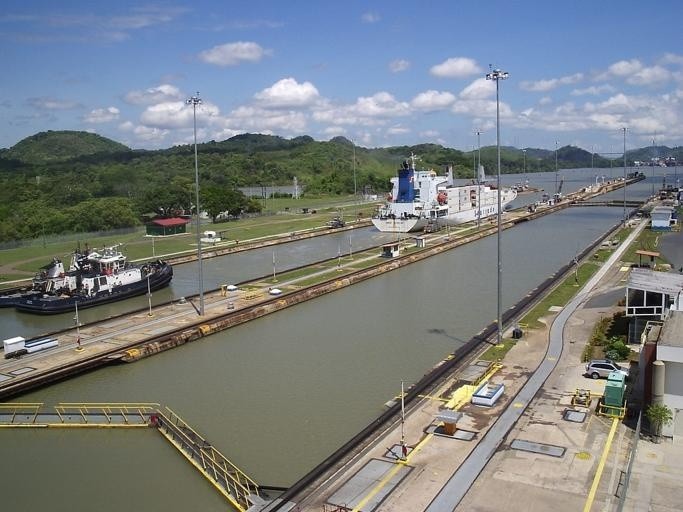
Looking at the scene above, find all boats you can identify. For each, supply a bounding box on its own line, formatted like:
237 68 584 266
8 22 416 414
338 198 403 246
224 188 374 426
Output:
0 284 45 308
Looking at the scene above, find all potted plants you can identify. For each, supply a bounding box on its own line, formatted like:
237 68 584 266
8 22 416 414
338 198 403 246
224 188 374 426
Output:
644 403 674 444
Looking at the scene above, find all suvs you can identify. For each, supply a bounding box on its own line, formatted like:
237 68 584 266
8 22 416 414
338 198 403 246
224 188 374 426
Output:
586 360 629 380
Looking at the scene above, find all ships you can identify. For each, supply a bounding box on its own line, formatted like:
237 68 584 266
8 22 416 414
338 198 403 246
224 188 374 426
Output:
370 149 517 234
16 240 173 317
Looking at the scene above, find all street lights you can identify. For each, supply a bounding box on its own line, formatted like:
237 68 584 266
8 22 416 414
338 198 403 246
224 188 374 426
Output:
186 95 205 315
472 127 486 227
485 71 508 347
552 138 560 194
618 126 630 224
519 143 528 184
